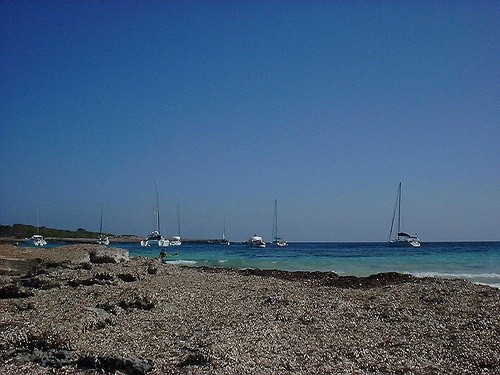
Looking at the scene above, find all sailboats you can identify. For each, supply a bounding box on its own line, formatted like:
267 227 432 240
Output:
140 174 170 247
387 181 421 248
96 203 110 246
272 198 288 247
220 216 230 246
170 200 183 246
28 204 47 247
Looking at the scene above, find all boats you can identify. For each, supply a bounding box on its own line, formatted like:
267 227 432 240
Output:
248 234 266 248
